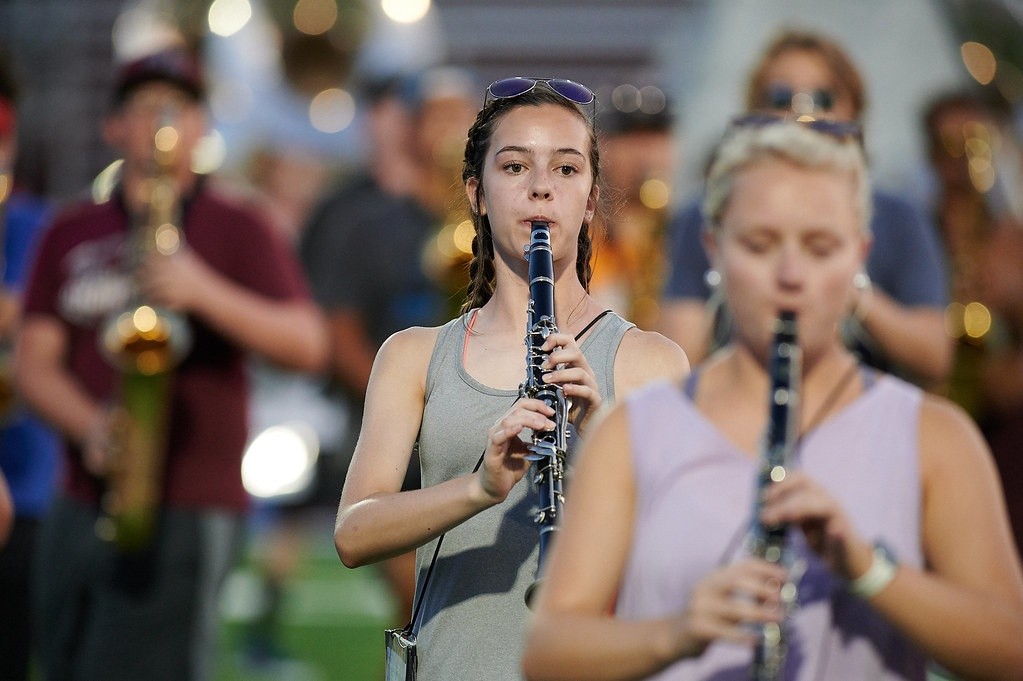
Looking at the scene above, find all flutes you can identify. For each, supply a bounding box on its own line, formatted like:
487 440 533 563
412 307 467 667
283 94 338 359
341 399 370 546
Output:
747 308 808 679
520 217 572 610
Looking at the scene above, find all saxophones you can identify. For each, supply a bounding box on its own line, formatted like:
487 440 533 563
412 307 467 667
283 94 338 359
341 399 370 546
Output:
92 124 190 556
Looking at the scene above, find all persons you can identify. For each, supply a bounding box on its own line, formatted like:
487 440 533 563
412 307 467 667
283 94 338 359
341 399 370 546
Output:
526 123 1023 681
656 33 948 388
918 89 1023 568
333 88 692 681
15 59 323 681
220 72 479 667
587 99 679 324
0 85 56 681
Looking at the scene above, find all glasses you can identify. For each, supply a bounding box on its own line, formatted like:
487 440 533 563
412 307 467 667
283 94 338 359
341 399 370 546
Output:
482 77 597 133
767 81 838 110
703 113 866 195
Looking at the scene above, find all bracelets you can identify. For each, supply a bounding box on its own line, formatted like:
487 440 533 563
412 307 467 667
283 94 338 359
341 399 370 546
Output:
841 554 896 602
